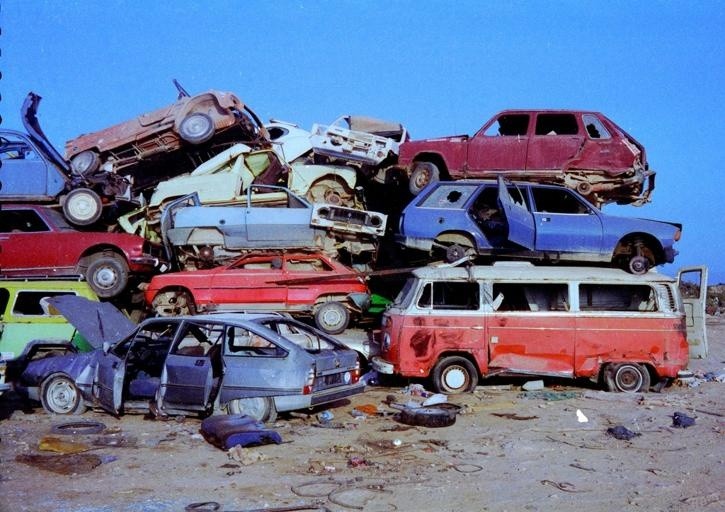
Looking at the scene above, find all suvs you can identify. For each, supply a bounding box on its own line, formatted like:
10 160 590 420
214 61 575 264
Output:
143 248 372 332
1 204 167 299
396 108 655 210
397 176 683 275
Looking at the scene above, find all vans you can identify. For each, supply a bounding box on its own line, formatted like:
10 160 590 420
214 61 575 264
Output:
380 259 690 395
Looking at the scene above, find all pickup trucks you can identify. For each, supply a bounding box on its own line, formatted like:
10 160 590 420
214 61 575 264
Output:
168 180 388 246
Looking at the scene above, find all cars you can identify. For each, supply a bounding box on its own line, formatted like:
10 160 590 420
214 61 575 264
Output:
21 297 365 425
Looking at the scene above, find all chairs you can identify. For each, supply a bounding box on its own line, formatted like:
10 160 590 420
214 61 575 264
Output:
128 347 204 396
